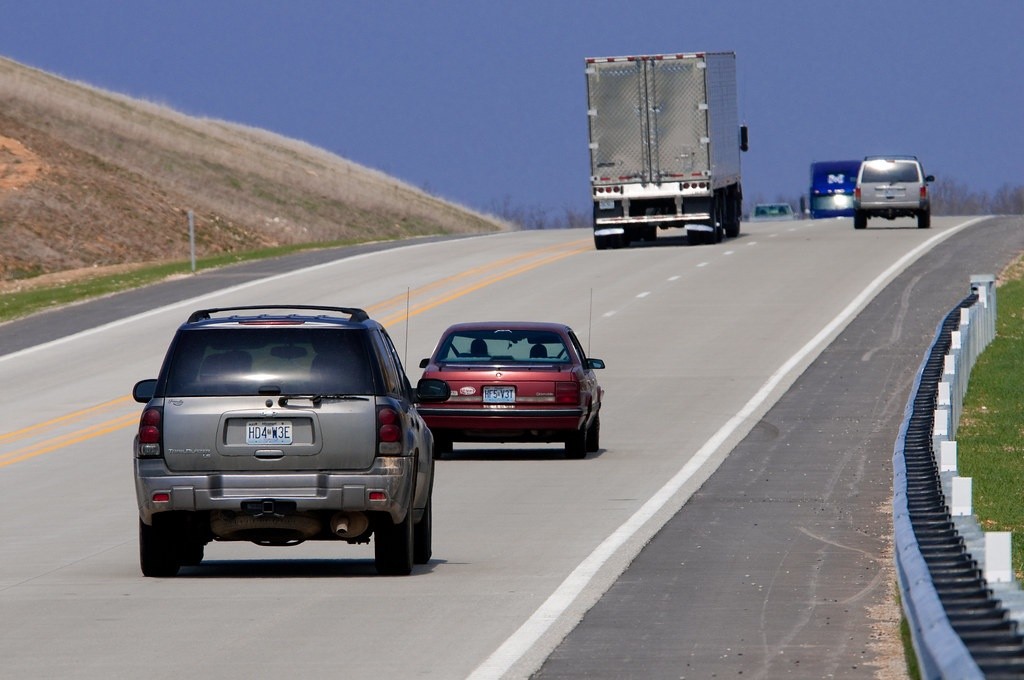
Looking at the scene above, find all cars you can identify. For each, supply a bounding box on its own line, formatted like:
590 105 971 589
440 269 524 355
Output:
410 319 606 460
750 203 796 223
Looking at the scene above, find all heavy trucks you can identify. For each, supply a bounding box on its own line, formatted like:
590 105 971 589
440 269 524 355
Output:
582 49 749 251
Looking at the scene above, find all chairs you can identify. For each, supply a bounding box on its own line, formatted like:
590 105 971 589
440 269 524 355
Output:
181 351 252 396
299 351 374 396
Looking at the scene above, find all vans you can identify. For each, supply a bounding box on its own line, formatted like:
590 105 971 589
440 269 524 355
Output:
808 159 865 222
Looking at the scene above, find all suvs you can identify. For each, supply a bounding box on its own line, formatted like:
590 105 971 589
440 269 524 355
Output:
133 303 452 579
852 154 937 230
